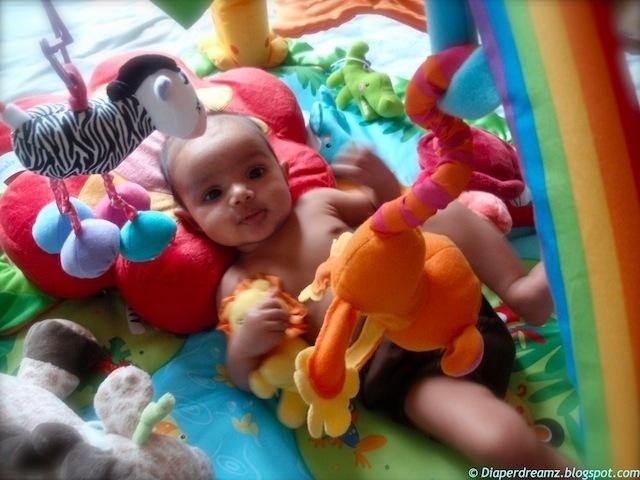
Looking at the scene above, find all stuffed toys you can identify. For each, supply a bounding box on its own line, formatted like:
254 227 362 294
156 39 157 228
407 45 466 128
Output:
0 43 533 480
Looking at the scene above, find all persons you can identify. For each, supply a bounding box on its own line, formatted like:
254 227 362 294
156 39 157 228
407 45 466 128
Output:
159 112 580 480
0 43 533 480
